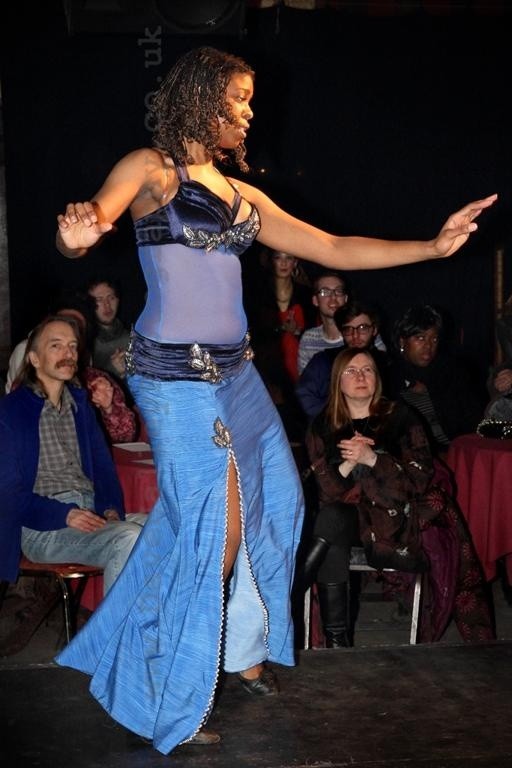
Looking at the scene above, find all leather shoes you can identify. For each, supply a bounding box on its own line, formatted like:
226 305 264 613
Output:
234 662 279 697
142 728 222 747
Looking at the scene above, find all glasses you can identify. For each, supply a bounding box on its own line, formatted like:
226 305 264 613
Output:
340 322 375 335
315 287 347 297
342 367 376 375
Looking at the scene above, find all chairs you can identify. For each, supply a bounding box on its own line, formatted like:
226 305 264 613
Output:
4 555 103 649
303 564 423 649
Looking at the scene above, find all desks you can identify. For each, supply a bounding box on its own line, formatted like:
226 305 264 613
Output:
446 431 511 577
64 438 157 612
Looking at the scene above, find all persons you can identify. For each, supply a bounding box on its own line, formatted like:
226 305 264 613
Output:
297 270 386 377
257 248 322 377
0 317 153 602
296 301 398 420
391 303 467 456
4 296 136 445
304 347 435 649
52 46 499 756
86 274 134 406
487 362 512 398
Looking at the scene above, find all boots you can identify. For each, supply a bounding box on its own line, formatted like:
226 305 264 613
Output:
315 580 350 650
289 535 327 605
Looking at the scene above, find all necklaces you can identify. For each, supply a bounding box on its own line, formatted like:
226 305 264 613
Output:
276 297 290 304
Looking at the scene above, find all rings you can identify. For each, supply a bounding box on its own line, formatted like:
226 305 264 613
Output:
349 450 353 456
